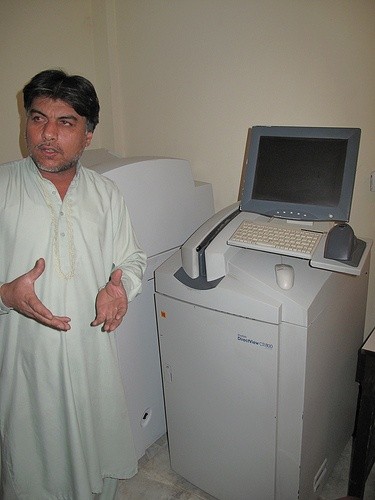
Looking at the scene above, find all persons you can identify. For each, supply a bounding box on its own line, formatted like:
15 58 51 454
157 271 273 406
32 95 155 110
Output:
0 69 149 500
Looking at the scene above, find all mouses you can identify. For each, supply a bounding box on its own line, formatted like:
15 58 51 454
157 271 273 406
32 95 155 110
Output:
275 264 295 290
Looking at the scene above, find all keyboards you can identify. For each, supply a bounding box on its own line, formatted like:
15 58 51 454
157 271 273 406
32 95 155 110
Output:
226 219 322 260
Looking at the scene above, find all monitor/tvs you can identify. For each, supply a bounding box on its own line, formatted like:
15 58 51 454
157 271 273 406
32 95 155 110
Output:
251 135 348 207
